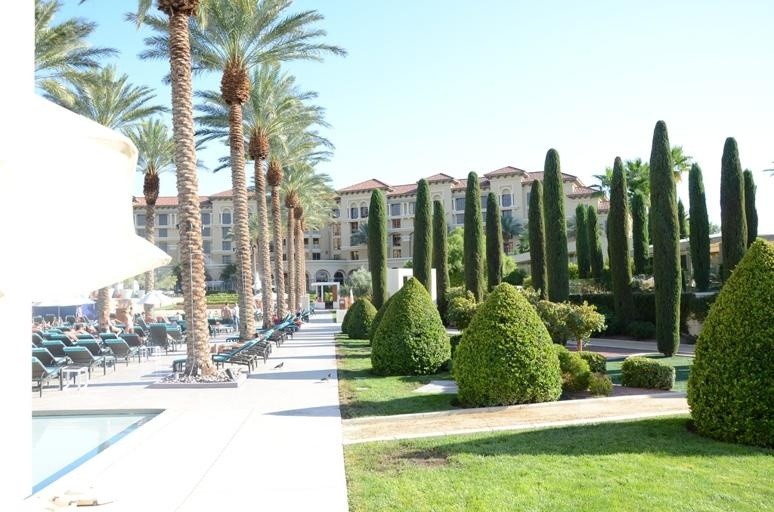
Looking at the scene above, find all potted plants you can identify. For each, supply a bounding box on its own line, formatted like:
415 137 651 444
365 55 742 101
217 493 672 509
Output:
324 291 334 309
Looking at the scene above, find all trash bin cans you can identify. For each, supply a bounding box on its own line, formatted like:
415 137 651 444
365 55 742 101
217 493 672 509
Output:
339 298 348 310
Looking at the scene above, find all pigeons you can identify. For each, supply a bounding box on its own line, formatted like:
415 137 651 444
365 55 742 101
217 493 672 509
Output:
274 362 284 369
321 373 333 383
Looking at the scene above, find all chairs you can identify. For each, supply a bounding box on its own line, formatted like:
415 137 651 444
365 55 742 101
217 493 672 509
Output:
31 314 297 398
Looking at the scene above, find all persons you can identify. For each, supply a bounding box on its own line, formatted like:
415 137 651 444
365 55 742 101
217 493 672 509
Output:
32 300 263 342
